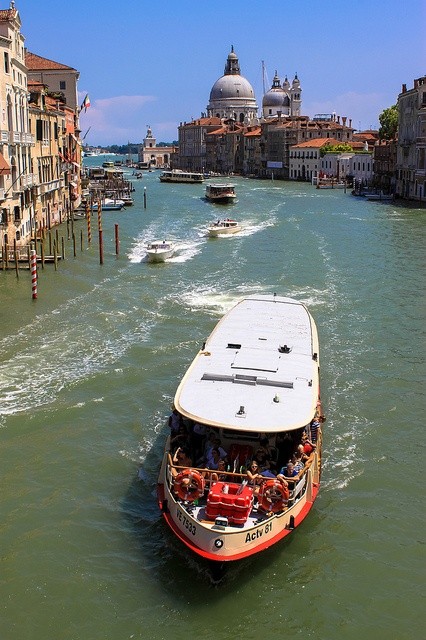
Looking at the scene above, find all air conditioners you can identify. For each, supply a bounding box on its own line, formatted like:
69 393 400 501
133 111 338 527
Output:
20 132 35 143
0 130 8 144
10 131 20 143
34 178 64 195
21 173 37 187
0 188 5 200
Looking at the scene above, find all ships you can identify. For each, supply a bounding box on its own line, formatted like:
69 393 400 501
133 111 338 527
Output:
155 168 206 184
153 292 324 584
204 183 237 204
136 161 148 170
101 161 114 168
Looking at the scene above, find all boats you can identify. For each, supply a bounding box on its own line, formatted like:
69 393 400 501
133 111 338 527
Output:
204 217 243 239
143 237 175 263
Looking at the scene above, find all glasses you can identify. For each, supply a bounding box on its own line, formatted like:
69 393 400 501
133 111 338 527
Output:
251 466 258 468
211 452 216 454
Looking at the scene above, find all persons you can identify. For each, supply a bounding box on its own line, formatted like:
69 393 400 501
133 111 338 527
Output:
312 419 320 444
276 462 300 496
223 444 238 472
243 461 264 485
252 449 278 478
297 426 317 455
173 446 192 474
171 424 191 457
276 431 293 466
260 438 282 476
168 404 183 440
192 420 206 459
216 220 223 227
289 457 303 470
292 445 313 466
197 440 228 468
205 448 223 470
201 460 226 487
289 452 305 467
196 432 216 465
